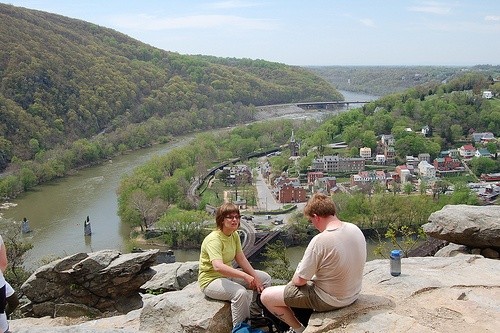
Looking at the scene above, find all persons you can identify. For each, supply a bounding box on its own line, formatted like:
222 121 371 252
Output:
0 234 19 333
198 203 271 328
260 192 367 333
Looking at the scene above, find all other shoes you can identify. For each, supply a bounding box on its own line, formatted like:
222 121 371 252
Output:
284 324 304 332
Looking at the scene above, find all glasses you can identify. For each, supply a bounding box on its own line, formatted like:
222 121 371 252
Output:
223 215 241 221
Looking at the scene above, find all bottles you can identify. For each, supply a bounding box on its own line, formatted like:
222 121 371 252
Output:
390 249 403 276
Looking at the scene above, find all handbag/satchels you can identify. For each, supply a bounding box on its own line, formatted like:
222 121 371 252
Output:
232 316 265 333
